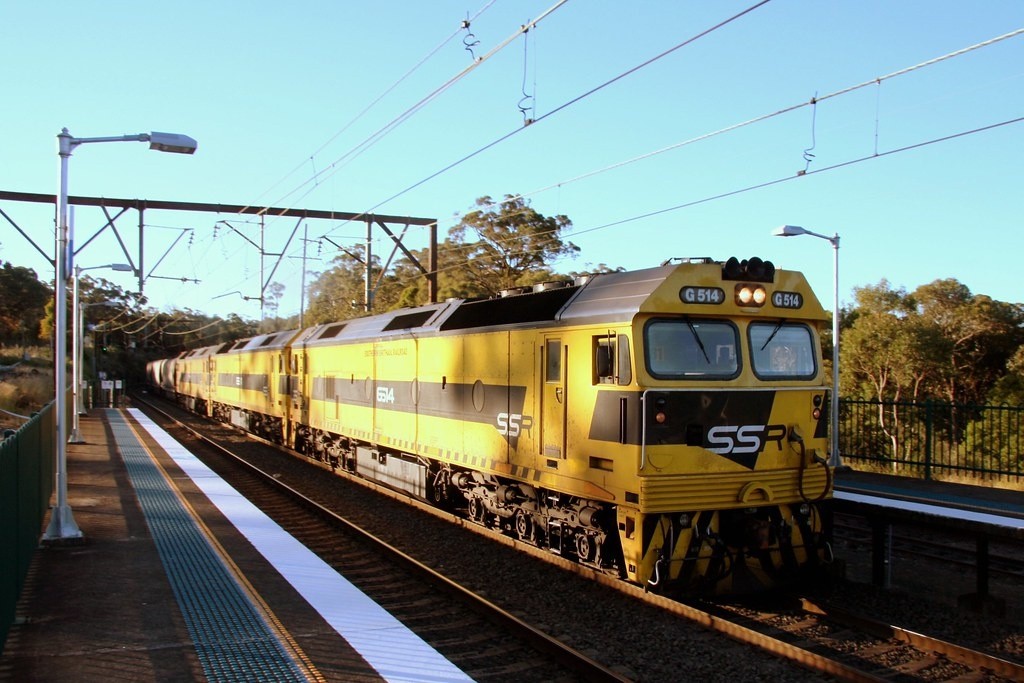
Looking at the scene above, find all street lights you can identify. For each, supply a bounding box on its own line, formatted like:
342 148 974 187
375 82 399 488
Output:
36 128 199 545
78 299 120 417
773 223 841 468
68 263 131 446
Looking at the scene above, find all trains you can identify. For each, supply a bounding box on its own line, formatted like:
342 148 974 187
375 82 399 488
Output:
142 253 835 590
